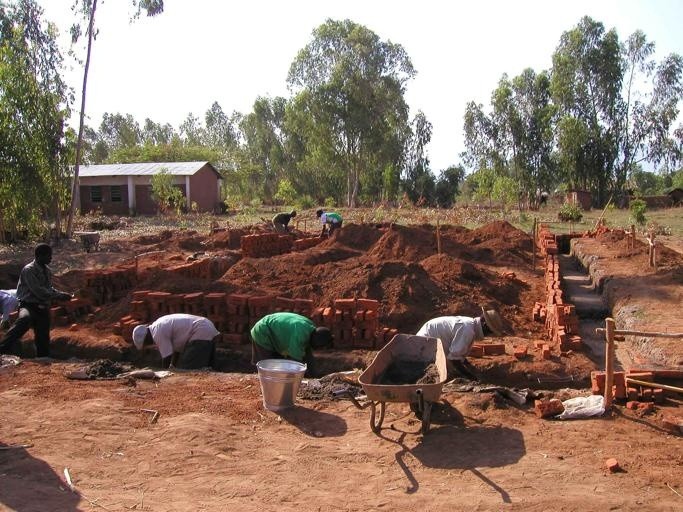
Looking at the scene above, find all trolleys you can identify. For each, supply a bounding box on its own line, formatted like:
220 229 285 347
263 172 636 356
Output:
326 331 451 439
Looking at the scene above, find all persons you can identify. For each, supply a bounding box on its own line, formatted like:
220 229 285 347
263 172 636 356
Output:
0 289 18 328
414 305 505 381
271 209 296 232
248 311 335 379
315 209 344 240
129 312 221 372
0 242 73 360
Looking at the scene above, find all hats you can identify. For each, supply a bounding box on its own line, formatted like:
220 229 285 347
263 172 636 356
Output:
481 306 504 339
132 324 150 349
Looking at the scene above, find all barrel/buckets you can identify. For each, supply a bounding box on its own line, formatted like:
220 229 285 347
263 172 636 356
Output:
255 359 308 411
